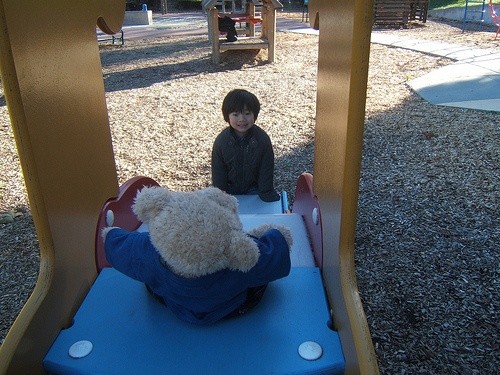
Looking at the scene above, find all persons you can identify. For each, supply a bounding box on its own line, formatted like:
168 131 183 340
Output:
227 21 239 42
211 89 281 203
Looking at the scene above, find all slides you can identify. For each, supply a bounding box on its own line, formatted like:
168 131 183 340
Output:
42 172 348 375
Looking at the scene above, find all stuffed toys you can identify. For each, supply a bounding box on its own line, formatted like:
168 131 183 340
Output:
99 186 292 324
